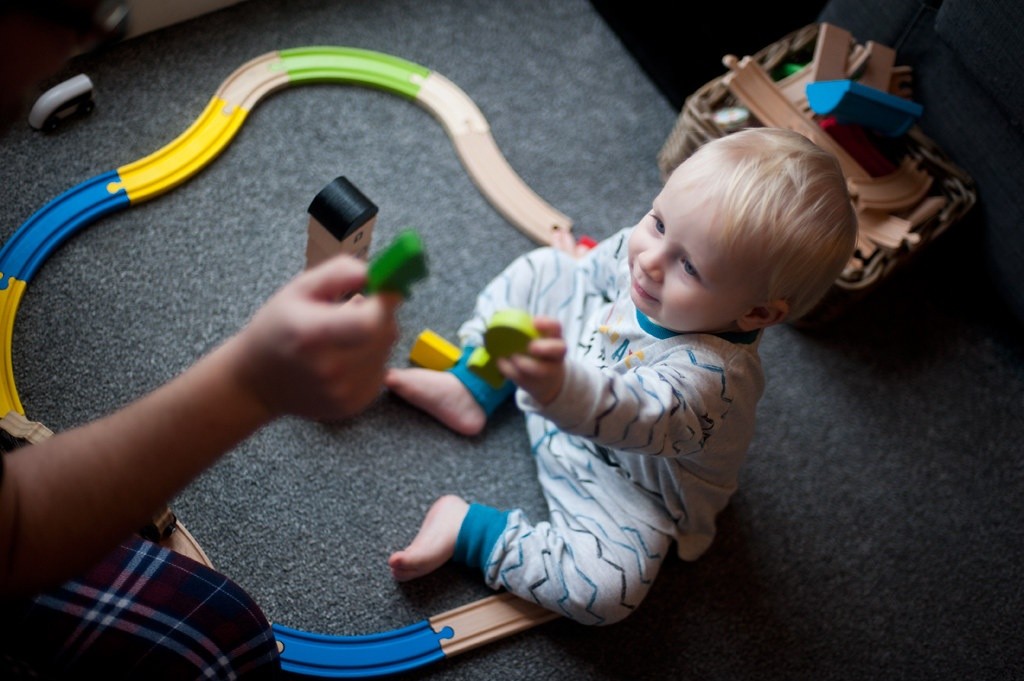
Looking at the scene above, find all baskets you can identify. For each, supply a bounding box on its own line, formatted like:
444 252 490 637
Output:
651 20 975 328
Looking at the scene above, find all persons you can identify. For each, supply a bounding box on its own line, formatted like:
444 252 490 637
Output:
0 252 401 681
384 127 857 624
